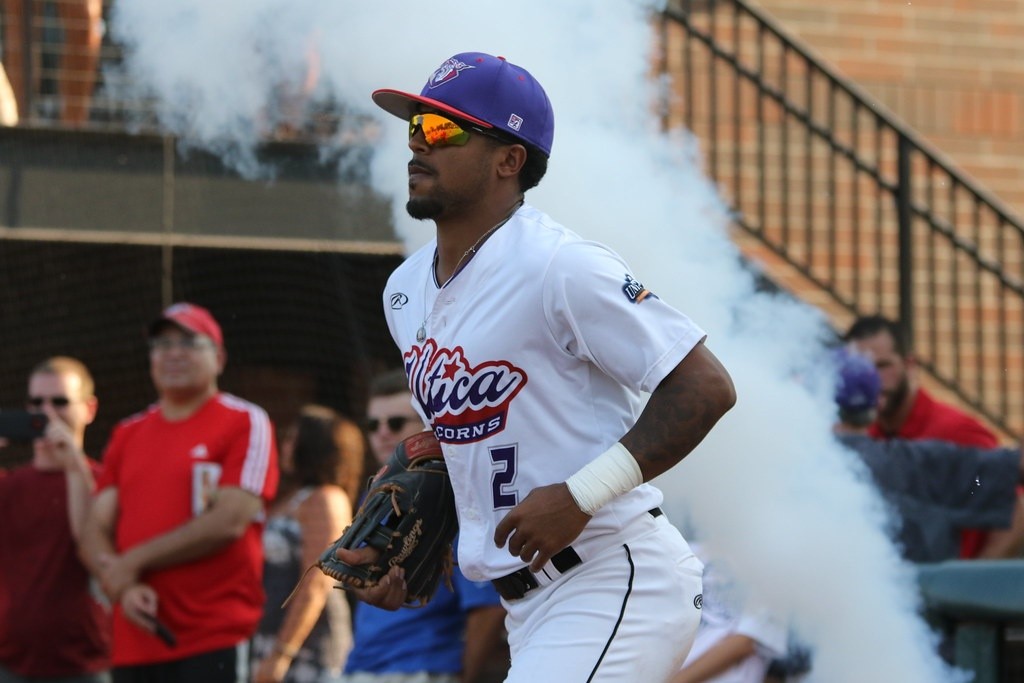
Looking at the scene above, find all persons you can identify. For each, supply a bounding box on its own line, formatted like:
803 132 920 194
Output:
673 537 791 683
78 304 279 683
244 406 364 683
0 357 102 683
834 316 1024 559
336 52 737 681
339 372 508 683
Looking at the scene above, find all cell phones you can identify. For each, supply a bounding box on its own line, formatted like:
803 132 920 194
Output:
138 610 177 650
0 412 51 441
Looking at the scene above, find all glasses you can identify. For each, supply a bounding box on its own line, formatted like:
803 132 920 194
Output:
147 333 211 352
363 416 422 436
408 114 517 148
25 395 81 410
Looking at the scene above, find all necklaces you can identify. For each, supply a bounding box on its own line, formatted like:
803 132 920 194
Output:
414 214 512 342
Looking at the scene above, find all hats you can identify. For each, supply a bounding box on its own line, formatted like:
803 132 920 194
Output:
830 345 880 409
150 302 225 355
371 52 555 158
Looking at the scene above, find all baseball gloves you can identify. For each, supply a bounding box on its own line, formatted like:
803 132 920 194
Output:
317 428 458 610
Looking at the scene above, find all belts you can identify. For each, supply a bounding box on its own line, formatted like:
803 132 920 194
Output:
491 508 662 600
